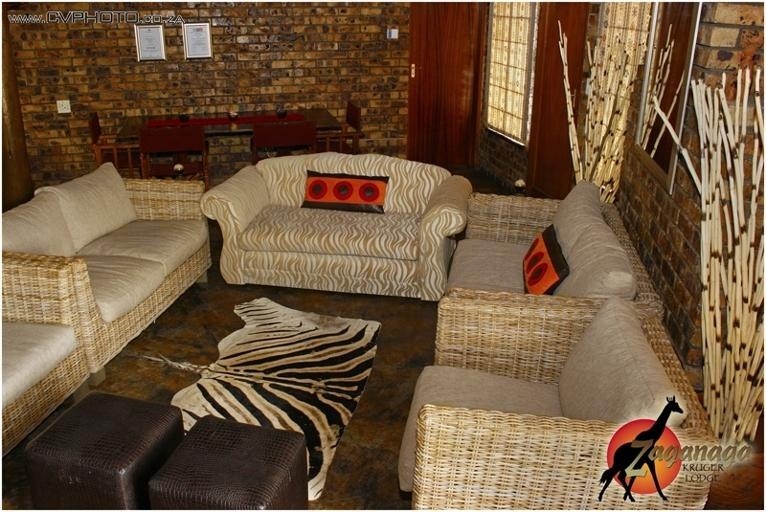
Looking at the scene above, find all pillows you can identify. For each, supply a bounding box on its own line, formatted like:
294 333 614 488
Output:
551 179 637 301
3 191 77 262
35 162 141 255
558 296 690 428
517 225 572 297
301 169 389 213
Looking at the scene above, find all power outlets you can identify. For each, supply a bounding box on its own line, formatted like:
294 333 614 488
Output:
56 99 72 113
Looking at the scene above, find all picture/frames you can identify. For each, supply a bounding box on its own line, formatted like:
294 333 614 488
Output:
181 21 214 60
133 22 167 63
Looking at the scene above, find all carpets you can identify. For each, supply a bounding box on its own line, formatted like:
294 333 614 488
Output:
168 294 383 502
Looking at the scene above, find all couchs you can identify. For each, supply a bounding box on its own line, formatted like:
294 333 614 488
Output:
3 257 91 460
396 298 724 510
3 161 214 386
437 179 669 305
200 149 472 306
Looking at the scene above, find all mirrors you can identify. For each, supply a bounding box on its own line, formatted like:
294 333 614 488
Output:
628 2 703 195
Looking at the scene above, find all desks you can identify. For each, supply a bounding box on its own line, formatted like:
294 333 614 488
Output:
115 107 343 178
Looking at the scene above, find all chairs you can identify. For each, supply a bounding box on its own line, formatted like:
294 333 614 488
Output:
251 120 317 165
88 111 142 179
138 126 210 192
317 101 365 153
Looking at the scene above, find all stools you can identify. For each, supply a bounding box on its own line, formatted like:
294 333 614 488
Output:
146 414 309 509
23 390 183 510
